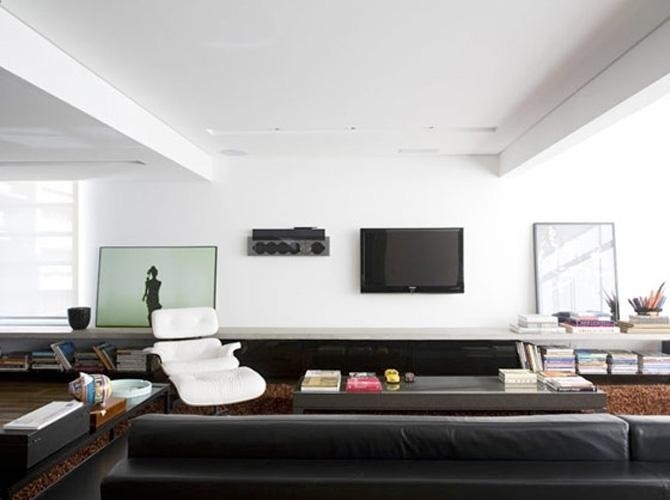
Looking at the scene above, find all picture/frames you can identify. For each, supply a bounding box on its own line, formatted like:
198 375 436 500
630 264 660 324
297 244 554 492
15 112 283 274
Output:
96 246 217 327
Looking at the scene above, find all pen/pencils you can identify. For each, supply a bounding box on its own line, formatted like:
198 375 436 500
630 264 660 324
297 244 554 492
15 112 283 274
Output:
628 281 666 311
603 290 619 314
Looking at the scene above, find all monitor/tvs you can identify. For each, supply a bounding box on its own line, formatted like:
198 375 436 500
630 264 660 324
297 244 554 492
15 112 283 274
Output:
360 227 465 293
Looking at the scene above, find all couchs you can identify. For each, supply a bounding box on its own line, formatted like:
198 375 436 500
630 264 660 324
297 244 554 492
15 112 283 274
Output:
30 413 670 500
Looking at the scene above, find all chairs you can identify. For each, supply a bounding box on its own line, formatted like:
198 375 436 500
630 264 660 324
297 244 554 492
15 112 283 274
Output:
142 307 267 415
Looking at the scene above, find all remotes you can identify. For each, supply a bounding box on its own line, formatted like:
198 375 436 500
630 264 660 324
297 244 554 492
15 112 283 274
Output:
405 371 414 383
385 368 399 383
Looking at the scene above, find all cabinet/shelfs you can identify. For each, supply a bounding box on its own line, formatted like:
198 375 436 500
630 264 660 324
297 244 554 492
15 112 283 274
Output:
0 329 670 384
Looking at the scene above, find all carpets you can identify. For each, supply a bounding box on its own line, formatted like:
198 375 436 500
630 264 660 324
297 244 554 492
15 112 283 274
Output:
9 385 670 500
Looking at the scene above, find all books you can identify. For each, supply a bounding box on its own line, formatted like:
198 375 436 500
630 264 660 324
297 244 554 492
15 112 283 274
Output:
345 372 383 392
300 369 341 392
1 340 147 373
499 310 670 392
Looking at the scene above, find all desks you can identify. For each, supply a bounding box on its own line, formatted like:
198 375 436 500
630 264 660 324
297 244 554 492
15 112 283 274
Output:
0 381 170 500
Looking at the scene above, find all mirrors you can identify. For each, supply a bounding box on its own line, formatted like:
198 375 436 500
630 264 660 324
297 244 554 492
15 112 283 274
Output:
533 223 619 321
